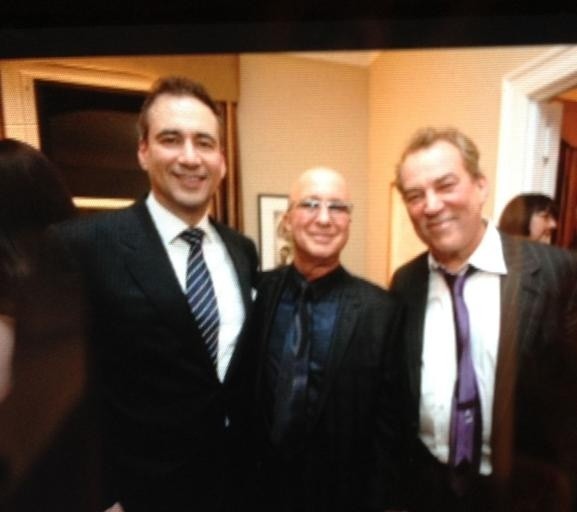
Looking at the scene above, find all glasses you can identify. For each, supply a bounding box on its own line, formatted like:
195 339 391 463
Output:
286 198 355 215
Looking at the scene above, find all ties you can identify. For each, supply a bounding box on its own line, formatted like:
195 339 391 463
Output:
261 281 320 476
433 263 483 476
178 226 221 377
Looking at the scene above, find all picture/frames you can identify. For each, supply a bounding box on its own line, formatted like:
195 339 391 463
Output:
257 193 295 273
386 179 429 292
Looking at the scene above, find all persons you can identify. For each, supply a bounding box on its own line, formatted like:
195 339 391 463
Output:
68 71 271 512
239 163 417 512
0 136 127 512
497 188 560 248
384 123 577 512
